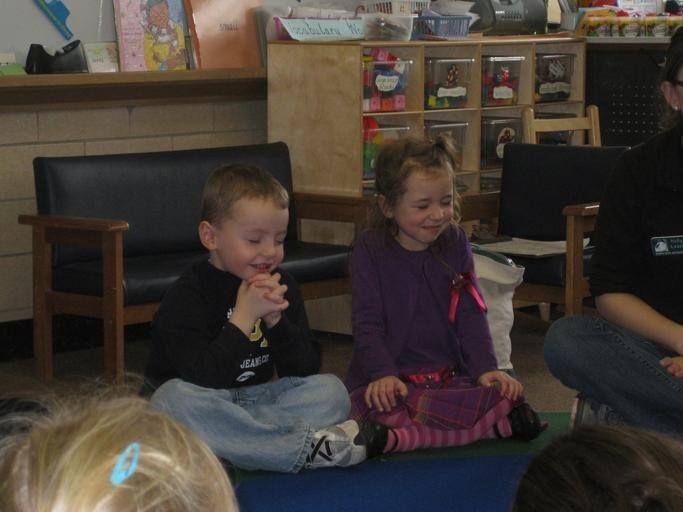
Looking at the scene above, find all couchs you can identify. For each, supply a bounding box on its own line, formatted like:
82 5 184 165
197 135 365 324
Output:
16 140 384 394
456 142 630 330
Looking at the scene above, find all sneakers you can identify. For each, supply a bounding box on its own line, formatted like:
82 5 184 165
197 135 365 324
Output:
304 418 369 470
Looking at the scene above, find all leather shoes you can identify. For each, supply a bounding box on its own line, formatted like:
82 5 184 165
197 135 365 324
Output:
360 415 398 458
492 403 541 441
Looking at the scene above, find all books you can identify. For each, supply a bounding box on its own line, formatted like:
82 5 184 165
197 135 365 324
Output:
112 0 193 73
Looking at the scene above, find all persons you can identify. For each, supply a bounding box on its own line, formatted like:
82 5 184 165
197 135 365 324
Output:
542 25 681 433
0 398 242 512
343 129 548 458
137 161 366 475
508 422 683 512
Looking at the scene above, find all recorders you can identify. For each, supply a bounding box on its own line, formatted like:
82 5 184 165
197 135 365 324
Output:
470 0 547 36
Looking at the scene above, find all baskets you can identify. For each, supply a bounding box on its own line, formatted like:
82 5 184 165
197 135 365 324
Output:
363 1 431 17
412 16 472 40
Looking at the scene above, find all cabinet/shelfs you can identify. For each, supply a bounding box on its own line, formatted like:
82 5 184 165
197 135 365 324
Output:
266 36 589 336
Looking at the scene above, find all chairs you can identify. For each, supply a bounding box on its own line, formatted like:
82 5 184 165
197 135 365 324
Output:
520 104 601 148
